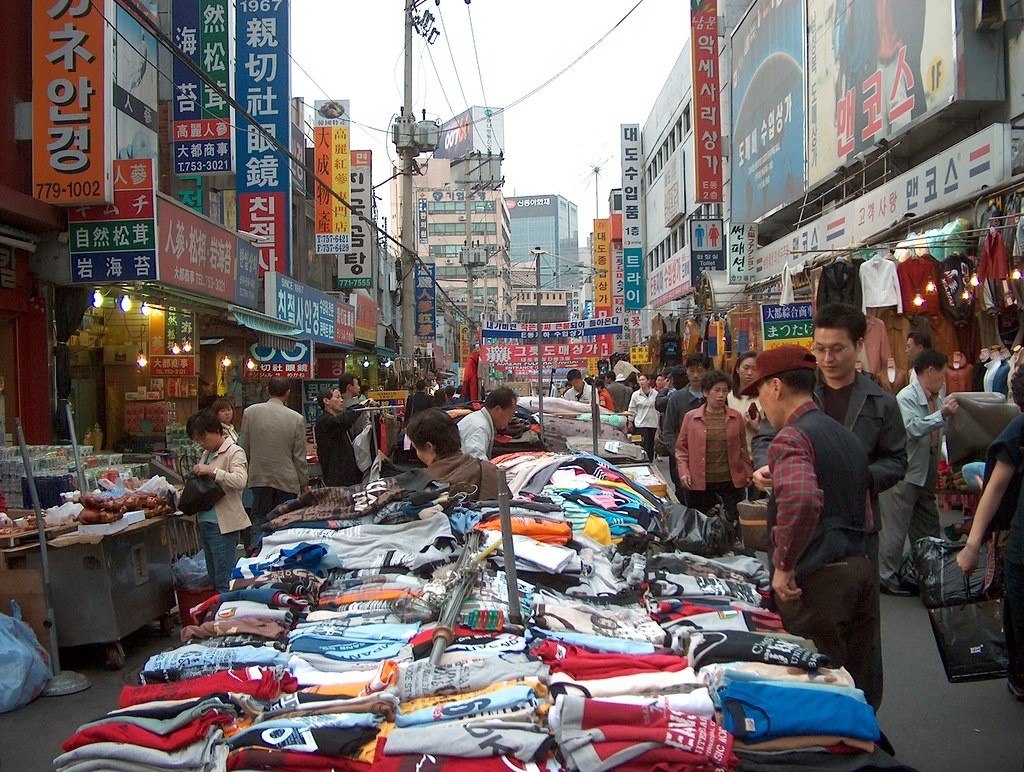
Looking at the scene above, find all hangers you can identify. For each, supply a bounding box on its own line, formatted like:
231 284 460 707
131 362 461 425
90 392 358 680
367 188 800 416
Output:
834 184 1024 265
431 479 478 560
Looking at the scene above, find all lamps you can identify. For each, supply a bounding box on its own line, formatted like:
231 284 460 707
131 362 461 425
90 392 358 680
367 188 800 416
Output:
834 165 846 175
874 138 887 150
853 151 864 162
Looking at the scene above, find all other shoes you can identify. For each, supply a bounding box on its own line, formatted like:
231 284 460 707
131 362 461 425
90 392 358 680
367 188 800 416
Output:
1008 680 1024 702
880 583 910 597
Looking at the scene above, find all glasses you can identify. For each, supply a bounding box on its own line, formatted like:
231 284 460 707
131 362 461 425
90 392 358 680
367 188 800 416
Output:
811 335 864 357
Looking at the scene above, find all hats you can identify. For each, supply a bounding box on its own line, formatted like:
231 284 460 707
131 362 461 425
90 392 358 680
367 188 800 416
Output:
739 345 816 396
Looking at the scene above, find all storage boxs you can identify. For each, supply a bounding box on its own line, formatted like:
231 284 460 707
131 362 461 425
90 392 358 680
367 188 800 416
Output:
124 378 192 444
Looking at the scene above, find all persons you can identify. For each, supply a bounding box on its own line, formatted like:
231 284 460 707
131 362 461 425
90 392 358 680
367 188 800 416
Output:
176 408 253 596
403 407 514 502
211 398 241 446
555 351 766 549
876 349 958 598
456 388 518 464
751 301 908 580
856 345 1024 413
739 343 883 717
314 373 486 489
904 331 947 462
954 362 1024 704
240 377 311 549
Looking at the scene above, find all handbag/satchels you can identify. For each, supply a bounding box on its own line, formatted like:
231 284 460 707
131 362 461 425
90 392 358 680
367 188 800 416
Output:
177 455 225 516
928 572 1011 684
